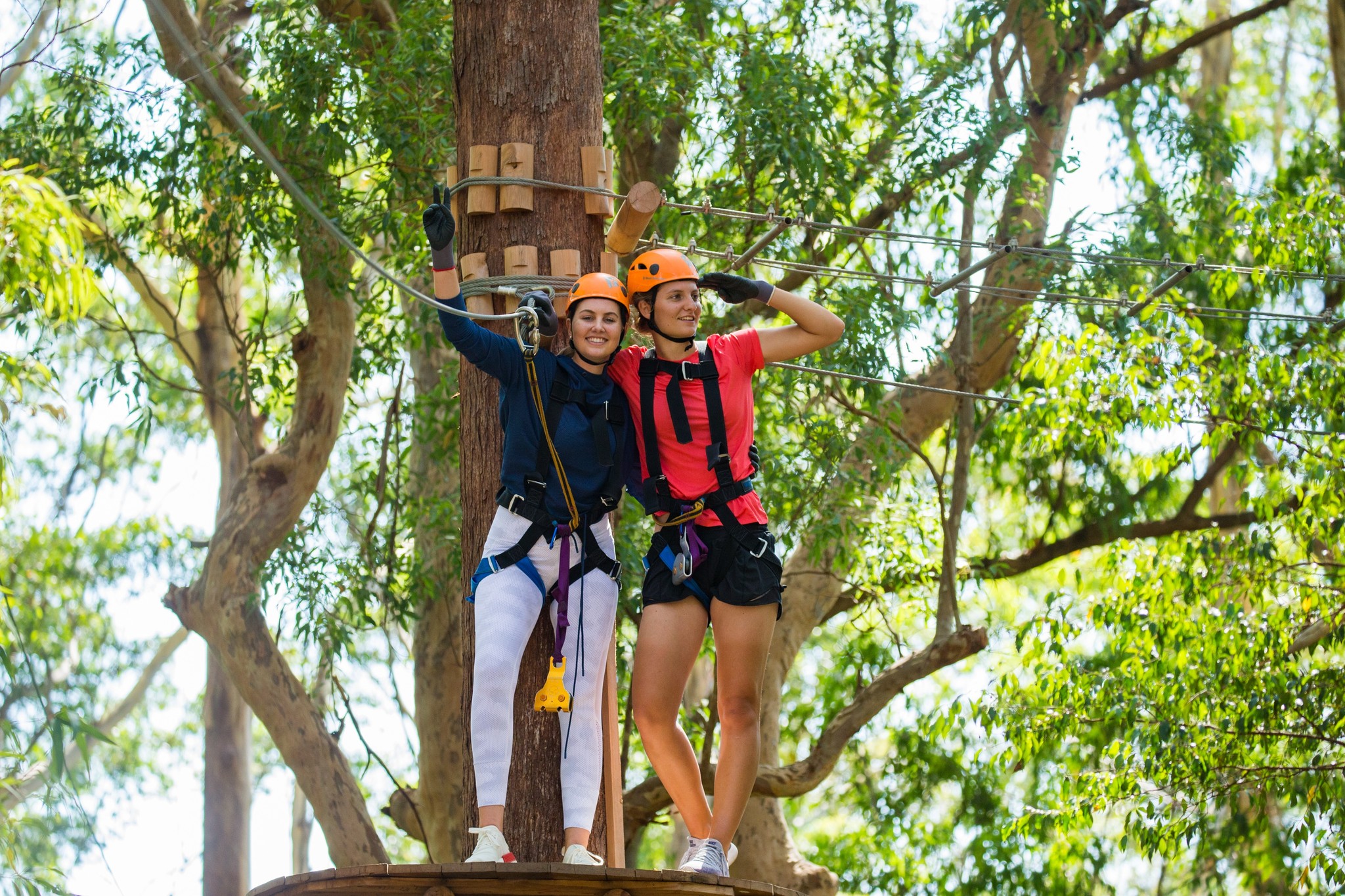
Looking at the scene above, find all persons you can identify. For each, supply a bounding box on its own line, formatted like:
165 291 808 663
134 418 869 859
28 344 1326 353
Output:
423 183 629 866
516 247 848 877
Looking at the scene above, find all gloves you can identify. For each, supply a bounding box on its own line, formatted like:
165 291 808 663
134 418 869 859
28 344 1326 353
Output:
696 272 776 305
423 184 456 272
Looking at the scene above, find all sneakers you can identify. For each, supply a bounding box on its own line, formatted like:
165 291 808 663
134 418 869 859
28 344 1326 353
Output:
679 838 730 878
464 825 518 863
677 836 739 871
562 844 604 866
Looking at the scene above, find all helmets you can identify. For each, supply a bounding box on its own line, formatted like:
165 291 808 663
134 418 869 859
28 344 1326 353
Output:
566 272 630 323
626 248 700 305
